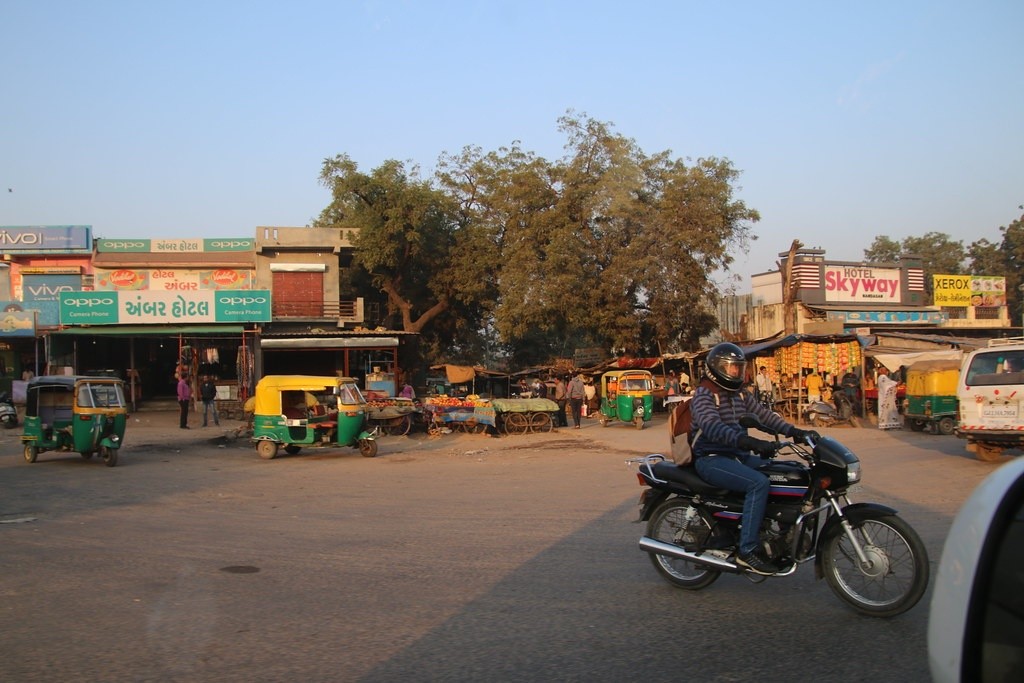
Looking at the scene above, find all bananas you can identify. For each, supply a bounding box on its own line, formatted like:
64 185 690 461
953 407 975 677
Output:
474 401 482 407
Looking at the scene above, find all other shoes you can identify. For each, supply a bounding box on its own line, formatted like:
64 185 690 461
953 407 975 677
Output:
182 426 189 429
574 424 579 427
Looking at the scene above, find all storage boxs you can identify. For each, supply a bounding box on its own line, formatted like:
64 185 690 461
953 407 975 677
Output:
214 386 238 400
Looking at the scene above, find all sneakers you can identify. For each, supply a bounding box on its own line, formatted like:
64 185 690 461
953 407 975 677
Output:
737 547 779 576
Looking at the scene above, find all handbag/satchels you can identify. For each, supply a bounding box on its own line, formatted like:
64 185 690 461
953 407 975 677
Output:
581 403 587 417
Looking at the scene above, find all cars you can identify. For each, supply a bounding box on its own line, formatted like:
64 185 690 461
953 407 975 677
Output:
958 336 1024 462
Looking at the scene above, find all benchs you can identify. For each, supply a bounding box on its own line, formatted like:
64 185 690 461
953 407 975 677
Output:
307 421 337 429
43 422 53 429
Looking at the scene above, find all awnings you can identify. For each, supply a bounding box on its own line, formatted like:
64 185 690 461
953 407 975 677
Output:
260 323 420 336
52 324 244 335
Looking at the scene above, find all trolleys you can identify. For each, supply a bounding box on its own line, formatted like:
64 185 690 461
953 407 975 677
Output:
366 411 554 436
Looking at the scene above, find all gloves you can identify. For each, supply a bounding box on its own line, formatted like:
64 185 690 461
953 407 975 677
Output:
737 433 775 461
788 426 820 445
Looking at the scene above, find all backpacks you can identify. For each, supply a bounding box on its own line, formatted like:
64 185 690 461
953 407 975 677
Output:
668 376 747 466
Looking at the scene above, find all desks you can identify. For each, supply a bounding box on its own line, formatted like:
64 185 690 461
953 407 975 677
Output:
423 402 494 434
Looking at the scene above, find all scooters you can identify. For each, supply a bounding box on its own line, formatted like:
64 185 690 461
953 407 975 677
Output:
759 390 783 418
805 389 859 428
0 390 19 429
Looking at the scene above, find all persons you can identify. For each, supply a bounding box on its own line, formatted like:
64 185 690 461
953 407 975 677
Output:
665 369 692 411
22 365 34 380
199 375 220 428
519 371 585 427
625 380 641 390
756 365 902 430
177 373 192 429
688 342 820 577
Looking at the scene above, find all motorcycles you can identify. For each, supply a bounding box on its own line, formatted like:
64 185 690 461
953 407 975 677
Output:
252 374 381 459
624 412 930 618
904 360 961 436
20 376 128 467
599 370 654 430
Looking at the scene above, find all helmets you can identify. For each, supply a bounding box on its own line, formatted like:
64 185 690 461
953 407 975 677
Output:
705 341 748 392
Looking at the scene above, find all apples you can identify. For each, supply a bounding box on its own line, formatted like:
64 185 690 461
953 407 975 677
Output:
439 397 473 407
